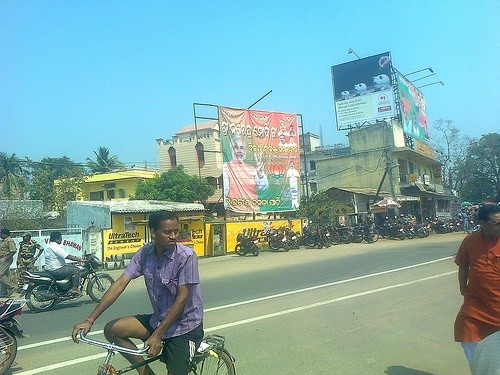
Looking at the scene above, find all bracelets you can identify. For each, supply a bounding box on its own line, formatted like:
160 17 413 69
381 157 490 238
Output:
87 317 94 324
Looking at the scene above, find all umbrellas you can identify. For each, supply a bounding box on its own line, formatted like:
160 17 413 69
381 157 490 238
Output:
375 198 401 217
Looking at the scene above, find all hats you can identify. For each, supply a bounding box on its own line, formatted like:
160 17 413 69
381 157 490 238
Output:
0 229 11 234
21 232 31 237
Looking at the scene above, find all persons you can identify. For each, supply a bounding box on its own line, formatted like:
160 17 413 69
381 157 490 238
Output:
16 232 45 294
223 137 269 213
454 204 500 375
72 209 204 375
0 227 17 297
280 122 300 207
45 231 88 294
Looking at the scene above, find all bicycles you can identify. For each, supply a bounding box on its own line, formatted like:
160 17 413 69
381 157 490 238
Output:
77 326 237 375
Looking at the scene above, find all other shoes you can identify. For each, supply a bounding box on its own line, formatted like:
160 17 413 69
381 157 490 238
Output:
71 291 83 296
0 289 7 295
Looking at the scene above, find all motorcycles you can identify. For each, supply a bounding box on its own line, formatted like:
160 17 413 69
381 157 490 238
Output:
0 298 30 375
265 206 483 252
234 228 261 256
20 251 115 312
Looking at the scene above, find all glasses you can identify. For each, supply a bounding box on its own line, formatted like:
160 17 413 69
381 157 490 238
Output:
487 220 500 226
